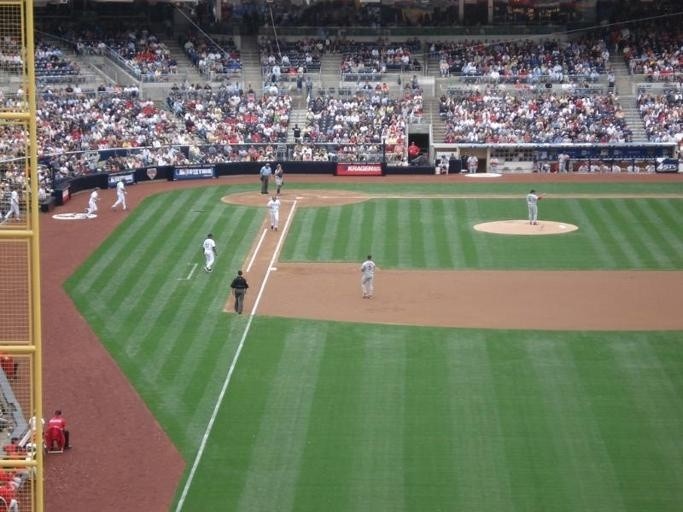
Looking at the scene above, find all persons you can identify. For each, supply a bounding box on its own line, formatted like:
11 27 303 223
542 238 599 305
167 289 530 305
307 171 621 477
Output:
359 256 376 299
1 346 50 510
202 234 217 273
230 270 249 314
6 186 23 222
86 187 101 214
526 189 547 225
1 0 682 206
44 409 70 452
112 178 129 210
